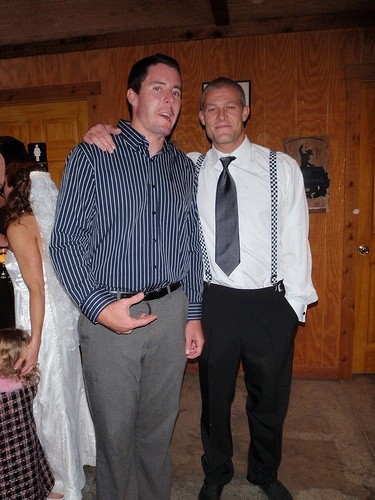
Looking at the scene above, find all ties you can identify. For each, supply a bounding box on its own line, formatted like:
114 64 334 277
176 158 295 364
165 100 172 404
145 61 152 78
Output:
215 157 240 277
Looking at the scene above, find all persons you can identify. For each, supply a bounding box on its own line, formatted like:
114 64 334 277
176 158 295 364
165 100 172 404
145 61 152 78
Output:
82 76 319 500
0 136 96 500
0 328 55 500
47 53 205 500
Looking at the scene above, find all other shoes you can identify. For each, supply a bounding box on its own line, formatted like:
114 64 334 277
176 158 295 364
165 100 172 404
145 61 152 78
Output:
197 470 234 500
247 473 293 500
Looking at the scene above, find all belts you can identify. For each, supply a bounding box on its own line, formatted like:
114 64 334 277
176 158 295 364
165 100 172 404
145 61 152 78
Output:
111 281 183 302
